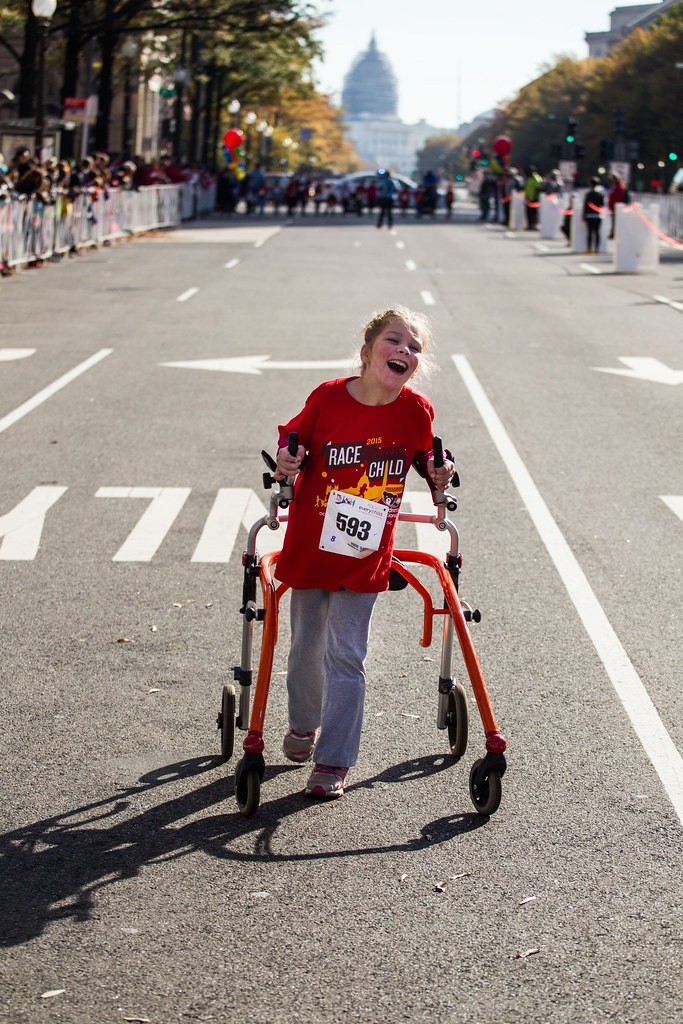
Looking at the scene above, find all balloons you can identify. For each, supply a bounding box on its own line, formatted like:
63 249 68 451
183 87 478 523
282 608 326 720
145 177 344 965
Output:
222 127 249 181
473 135 512 174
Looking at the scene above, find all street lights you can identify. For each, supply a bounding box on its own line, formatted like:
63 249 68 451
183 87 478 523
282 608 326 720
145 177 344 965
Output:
254 120 299 173
173 67 186 164
33 0 57 158
227 98 241 127
243 112 258 170
121 37 137 162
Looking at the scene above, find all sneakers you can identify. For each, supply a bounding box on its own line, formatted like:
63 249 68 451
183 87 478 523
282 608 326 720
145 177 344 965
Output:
305 765 348 798
282 728 315 762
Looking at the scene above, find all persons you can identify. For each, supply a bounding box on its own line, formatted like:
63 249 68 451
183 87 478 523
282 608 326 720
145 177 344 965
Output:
608 171 624 239
0 146 524 268
524 164 543 230
544 169 564 195
273 308 456 797
581 177 605 255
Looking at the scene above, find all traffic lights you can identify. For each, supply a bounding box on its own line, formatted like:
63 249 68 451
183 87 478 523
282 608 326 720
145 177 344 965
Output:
566 118 578 144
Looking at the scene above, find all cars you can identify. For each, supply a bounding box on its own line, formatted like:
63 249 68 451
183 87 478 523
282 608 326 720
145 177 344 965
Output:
324 172 447 208
